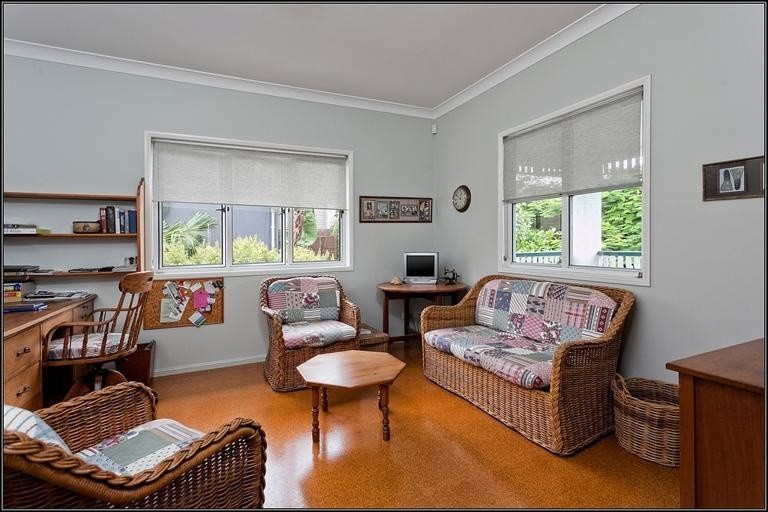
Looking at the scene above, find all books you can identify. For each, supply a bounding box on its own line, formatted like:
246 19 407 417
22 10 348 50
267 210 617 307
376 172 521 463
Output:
4 281 89 313
96 205 138 234
3 264 138 275
3 222 51 236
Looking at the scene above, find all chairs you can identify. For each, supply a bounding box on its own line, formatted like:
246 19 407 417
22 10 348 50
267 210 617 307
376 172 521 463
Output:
258 273 360 394
42 271 154 402
2 382 266 509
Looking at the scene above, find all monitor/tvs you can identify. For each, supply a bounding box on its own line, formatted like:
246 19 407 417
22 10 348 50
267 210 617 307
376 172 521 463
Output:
403 252 439 284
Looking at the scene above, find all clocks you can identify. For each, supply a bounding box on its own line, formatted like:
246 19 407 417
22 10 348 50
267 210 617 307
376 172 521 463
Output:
451 186 470 213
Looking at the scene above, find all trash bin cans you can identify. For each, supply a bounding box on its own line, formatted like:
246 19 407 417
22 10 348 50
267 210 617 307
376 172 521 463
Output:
115 339 156 387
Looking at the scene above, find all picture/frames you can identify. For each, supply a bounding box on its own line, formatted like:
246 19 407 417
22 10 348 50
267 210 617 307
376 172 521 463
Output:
359 194 433 225
700 154 763 203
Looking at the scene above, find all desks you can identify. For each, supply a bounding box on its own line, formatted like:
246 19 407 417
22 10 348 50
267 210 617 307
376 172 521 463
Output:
376 281 466 337
2 294 97 413
665 339 764 509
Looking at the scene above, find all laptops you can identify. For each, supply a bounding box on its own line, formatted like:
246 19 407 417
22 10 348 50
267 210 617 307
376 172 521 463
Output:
4 302 44 313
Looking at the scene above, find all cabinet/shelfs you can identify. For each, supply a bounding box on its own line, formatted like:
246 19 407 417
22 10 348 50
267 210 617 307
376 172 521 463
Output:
4 177 144 281
4 325 42 414
71 301 93 335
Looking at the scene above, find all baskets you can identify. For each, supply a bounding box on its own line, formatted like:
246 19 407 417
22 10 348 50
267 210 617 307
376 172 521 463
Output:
610 373 679 468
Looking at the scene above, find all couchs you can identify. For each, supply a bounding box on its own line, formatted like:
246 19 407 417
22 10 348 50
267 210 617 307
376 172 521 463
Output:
420 274 635 458
